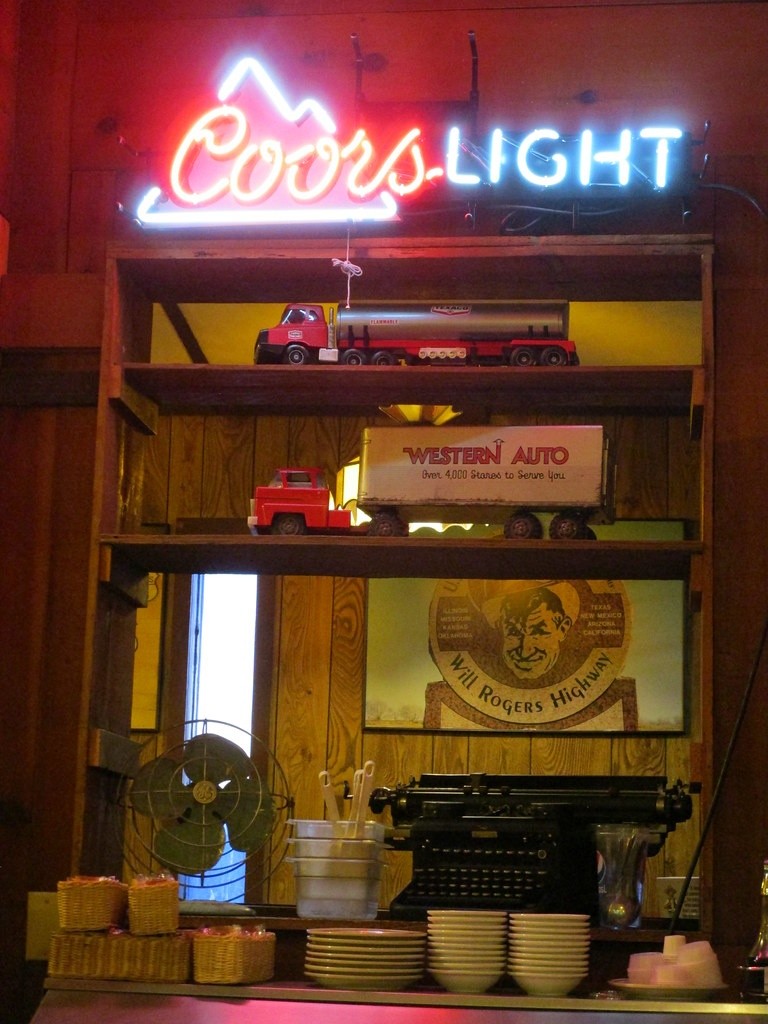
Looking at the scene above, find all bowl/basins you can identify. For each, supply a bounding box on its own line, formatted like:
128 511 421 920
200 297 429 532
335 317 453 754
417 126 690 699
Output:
628 941 724 988
509 914 590 996
427 910 508 992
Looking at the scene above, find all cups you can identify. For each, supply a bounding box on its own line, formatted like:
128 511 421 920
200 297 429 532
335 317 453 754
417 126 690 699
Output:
656 877 700 918
662 935 685 963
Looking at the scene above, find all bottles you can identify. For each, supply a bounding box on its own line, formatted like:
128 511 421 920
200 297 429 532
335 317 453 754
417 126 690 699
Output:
746 859 768 1003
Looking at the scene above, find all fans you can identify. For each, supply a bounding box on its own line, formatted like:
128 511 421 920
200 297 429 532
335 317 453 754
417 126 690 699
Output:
113 720 293 915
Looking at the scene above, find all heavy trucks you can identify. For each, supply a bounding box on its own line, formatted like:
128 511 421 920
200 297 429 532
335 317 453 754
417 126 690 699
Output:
253 298 582 367
246 425 619 541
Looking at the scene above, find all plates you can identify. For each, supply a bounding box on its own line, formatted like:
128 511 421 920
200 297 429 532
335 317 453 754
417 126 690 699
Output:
610 978 729 1000
305 927 427 991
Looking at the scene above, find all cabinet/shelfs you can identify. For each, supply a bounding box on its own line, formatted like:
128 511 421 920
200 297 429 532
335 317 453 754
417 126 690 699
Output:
70 235 714 997
